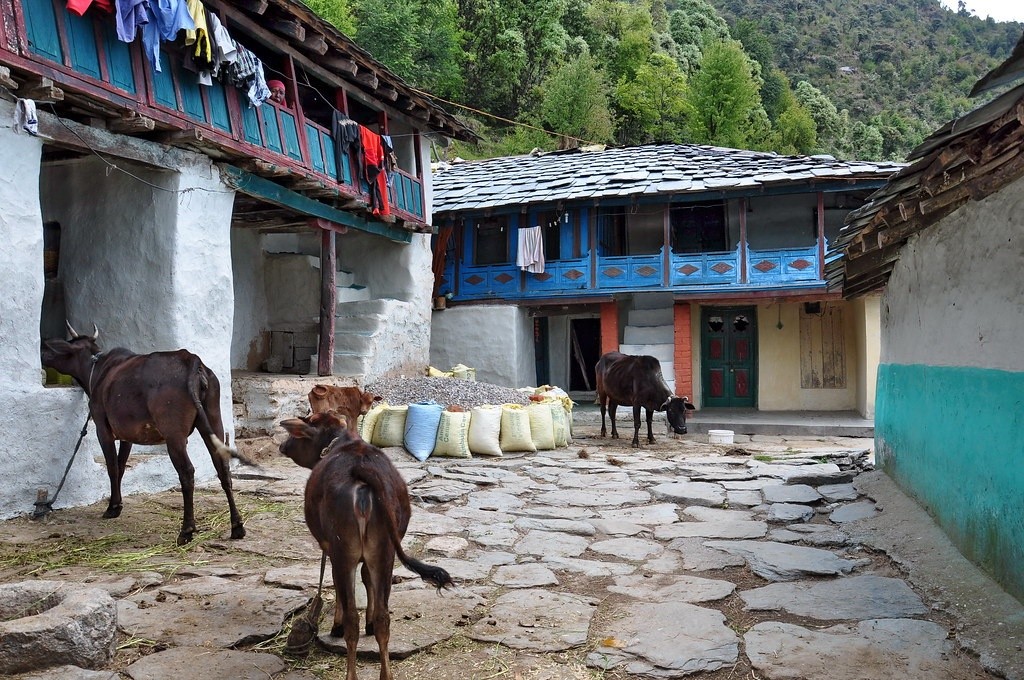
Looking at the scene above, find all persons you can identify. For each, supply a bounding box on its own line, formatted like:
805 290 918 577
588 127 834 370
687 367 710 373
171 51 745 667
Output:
268 79 287 107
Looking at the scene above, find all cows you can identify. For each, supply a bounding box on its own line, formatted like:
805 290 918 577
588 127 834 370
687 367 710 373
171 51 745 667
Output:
595 350 696 449
278 412 454 680
39 318 247 546
307 384 383 436
